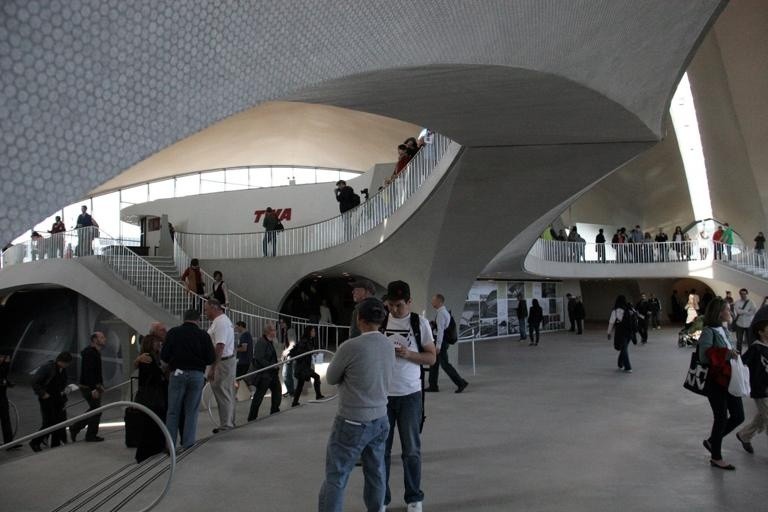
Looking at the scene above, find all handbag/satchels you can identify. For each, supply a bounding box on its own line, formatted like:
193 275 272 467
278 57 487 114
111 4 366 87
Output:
729 321 738 333
443 312 458 345
276 219 283 233
243 361 264 387
614 324 625 350
683 351 716 396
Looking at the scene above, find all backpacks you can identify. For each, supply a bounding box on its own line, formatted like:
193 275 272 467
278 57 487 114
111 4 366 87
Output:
352 193 360 210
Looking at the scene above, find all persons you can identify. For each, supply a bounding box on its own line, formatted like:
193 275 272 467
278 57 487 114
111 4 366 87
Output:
735 318 768 456
168 222 174 243
315 297 398 512
181 257 230 311
2 205 94 259
0 332 107 452
263 207 277 257
334 180 354 241
286 273 469 393
371 277 437 511
133 299 326 465
509 219 768 373
377 130 438 217
696 297 745 470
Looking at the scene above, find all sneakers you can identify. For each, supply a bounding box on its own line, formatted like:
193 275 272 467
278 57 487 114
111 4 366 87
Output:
28 426 76 452
407 501 422 512
283 391 293 398
87 437 103 442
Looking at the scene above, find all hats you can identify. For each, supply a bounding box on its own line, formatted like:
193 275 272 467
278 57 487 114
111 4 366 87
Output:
358 298 385 320
386 279 410 299
348 279 376 295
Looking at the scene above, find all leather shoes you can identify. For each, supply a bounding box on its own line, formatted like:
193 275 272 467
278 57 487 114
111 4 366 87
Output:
455 380 468 393
702 440 735 470
736 431 753 453
425 386 438 392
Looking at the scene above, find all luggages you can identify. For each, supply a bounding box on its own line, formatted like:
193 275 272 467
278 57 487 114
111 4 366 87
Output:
125 376 139 447
